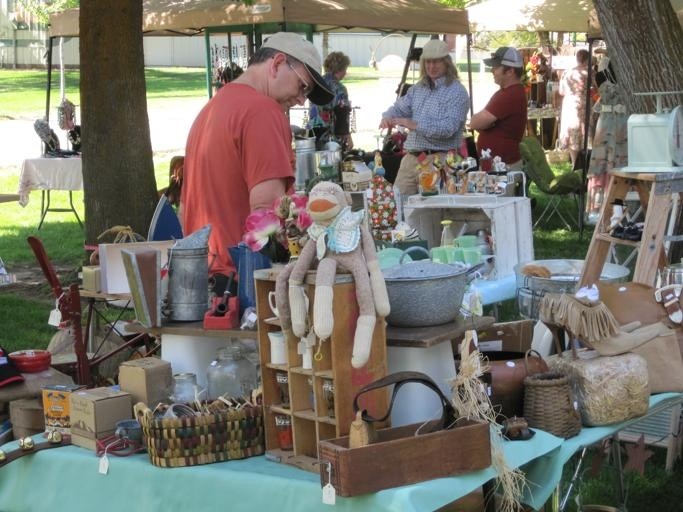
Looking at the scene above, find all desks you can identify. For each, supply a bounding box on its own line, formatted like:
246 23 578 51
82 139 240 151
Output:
25 155 89 231
540 390 683 512
122 301 494 433
5 405 540 512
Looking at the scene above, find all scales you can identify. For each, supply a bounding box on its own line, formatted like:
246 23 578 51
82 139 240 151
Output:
620 92 683 174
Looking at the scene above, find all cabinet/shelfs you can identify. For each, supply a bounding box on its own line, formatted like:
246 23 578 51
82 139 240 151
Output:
253 268 388 476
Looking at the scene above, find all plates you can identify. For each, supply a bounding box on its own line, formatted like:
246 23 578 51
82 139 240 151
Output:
378 249 412 268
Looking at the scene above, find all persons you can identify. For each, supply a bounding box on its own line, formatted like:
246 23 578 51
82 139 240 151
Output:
559 49 599 171
471 47 528 198
309 52 354 153
220 62 243 84
378 39 470 196
178 31 334 281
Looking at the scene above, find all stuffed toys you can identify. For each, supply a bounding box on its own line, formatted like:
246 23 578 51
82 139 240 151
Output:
275 180 391 369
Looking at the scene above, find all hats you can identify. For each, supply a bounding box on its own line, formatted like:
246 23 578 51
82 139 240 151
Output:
261 31 335 106
421 39 449 58
483 47 523 67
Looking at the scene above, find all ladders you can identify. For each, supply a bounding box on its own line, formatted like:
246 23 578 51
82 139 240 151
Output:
575 172 683 291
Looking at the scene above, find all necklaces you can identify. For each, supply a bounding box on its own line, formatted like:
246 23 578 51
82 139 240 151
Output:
34 101 82 159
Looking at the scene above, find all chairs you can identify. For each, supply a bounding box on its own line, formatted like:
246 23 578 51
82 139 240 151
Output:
522 137 583 232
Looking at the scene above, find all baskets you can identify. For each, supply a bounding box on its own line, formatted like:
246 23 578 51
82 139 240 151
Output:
140 396 265 468
524 350 581 439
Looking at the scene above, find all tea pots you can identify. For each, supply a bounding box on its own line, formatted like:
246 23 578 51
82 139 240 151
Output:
227 242 273 319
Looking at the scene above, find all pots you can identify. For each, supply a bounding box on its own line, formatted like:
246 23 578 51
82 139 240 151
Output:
385 246 468 327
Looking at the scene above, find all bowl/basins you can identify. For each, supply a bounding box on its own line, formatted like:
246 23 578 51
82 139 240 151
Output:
8 350 52 372
114 420 141 440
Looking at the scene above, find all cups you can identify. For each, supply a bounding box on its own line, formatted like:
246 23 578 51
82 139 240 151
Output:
276 414 292 451
323 382 334 419
444 248 454 264
463 248 481 265
454 235 475 247
430 246 444 263
276 372 290 409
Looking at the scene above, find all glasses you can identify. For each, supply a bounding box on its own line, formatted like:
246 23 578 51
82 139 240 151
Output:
271 55 312 96
